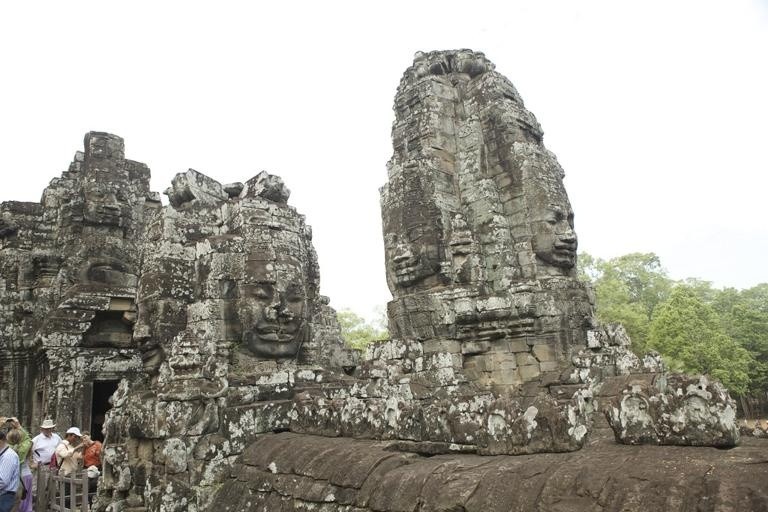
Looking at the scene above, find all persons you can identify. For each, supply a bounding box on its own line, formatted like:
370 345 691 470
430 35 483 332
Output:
49 426 85 508
31 418 63 512
379 169 443 285
0 430 20 512
517 144 578 268
79 431 102 503
0 417 33 511
80 161 137 268
21 440 34 511
234 228 309 358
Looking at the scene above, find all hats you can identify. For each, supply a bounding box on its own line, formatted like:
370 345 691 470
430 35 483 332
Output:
40 419 56 429
86 466 100 479
66 428 82 436
